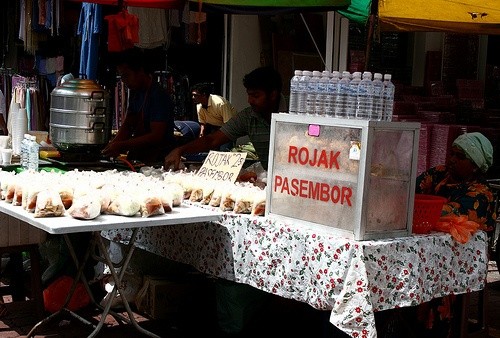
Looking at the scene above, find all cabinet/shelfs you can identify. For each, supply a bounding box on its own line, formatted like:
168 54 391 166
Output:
265 113 425 239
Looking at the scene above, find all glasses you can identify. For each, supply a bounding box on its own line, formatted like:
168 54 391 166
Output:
449 146 467 160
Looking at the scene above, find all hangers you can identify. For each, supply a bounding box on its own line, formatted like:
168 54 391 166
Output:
12 71 40 95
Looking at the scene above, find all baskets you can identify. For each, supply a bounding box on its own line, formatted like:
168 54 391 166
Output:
413 193 448 233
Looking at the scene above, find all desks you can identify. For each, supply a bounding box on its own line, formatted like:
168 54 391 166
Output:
0 175 492 338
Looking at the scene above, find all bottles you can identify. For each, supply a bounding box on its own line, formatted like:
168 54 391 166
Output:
20 133 39 171
289 70 395 123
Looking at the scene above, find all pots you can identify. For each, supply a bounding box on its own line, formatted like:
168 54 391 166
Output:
48 73 113 145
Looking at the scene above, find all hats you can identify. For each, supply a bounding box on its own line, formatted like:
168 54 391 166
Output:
454 132 493 173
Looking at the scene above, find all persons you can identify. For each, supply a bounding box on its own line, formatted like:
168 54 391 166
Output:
162 64 297 185
105 50 175 169
187 85 243 162
399 132 497 338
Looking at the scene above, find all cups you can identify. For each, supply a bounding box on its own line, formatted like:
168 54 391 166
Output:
1 149 13 165
